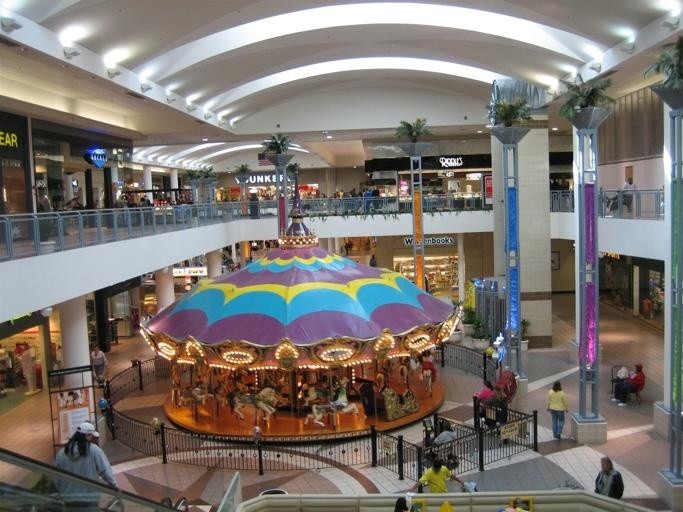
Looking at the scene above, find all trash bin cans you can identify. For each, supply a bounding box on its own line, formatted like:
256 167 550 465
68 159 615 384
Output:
258 489 288 496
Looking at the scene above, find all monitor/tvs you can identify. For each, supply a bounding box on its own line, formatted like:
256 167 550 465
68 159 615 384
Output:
414 179 427 186
428 180 442 186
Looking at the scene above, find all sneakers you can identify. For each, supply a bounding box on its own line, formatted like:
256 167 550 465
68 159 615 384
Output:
611 398 621 402
618 403 627 407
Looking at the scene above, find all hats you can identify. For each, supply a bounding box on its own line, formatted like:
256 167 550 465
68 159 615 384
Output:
396 498 408 511
634 364 642 369
76 422 100 437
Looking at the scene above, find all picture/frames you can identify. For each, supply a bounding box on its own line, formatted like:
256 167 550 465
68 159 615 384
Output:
551 251 560 271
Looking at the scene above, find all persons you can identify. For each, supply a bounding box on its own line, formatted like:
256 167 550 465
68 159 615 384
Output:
91 345 108 377
321 350 521 512
54 421 119 507
546 381 568 440
21 345 34 391
595 458 624 498
623 175 639 213
610 363 645 407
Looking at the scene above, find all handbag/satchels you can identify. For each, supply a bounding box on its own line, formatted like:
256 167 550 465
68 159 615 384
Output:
617 366 629 379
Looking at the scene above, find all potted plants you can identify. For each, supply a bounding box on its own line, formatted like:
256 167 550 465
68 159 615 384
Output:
519 319 530 352
460 305 475 335
470 318 491 353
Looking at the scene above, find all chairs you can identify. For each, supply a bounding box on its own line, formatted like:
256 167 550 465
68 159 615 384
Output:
628 381 644 407
610 363 627 394
